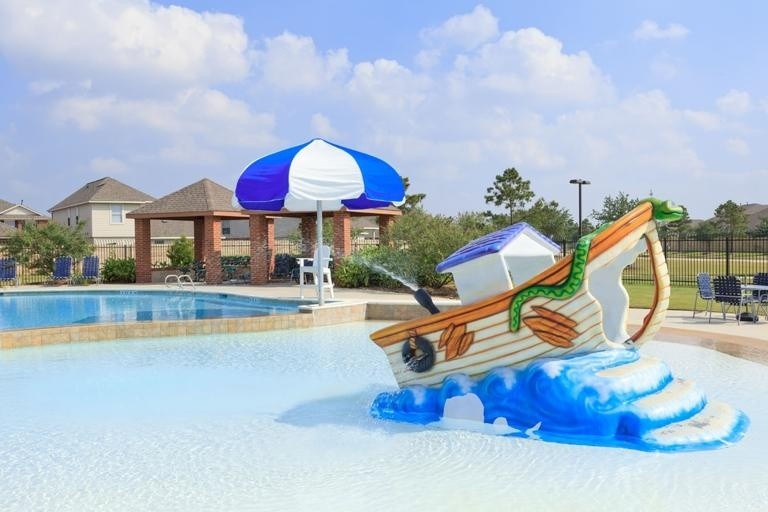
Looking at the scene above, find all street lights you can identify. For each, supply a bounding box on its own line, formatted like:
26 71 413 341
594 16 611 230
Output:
569 179 591 239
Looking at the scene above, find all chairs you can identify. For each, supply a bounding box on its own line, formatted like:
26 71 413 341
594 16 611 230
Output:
50 254 73 286
0 256 18 289
79 254 101 287
296 245 336 306
693 271 766 328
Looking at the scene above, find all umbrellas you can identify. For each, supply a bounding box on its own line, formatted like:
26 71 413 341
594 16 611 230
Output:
234 136 407 305
